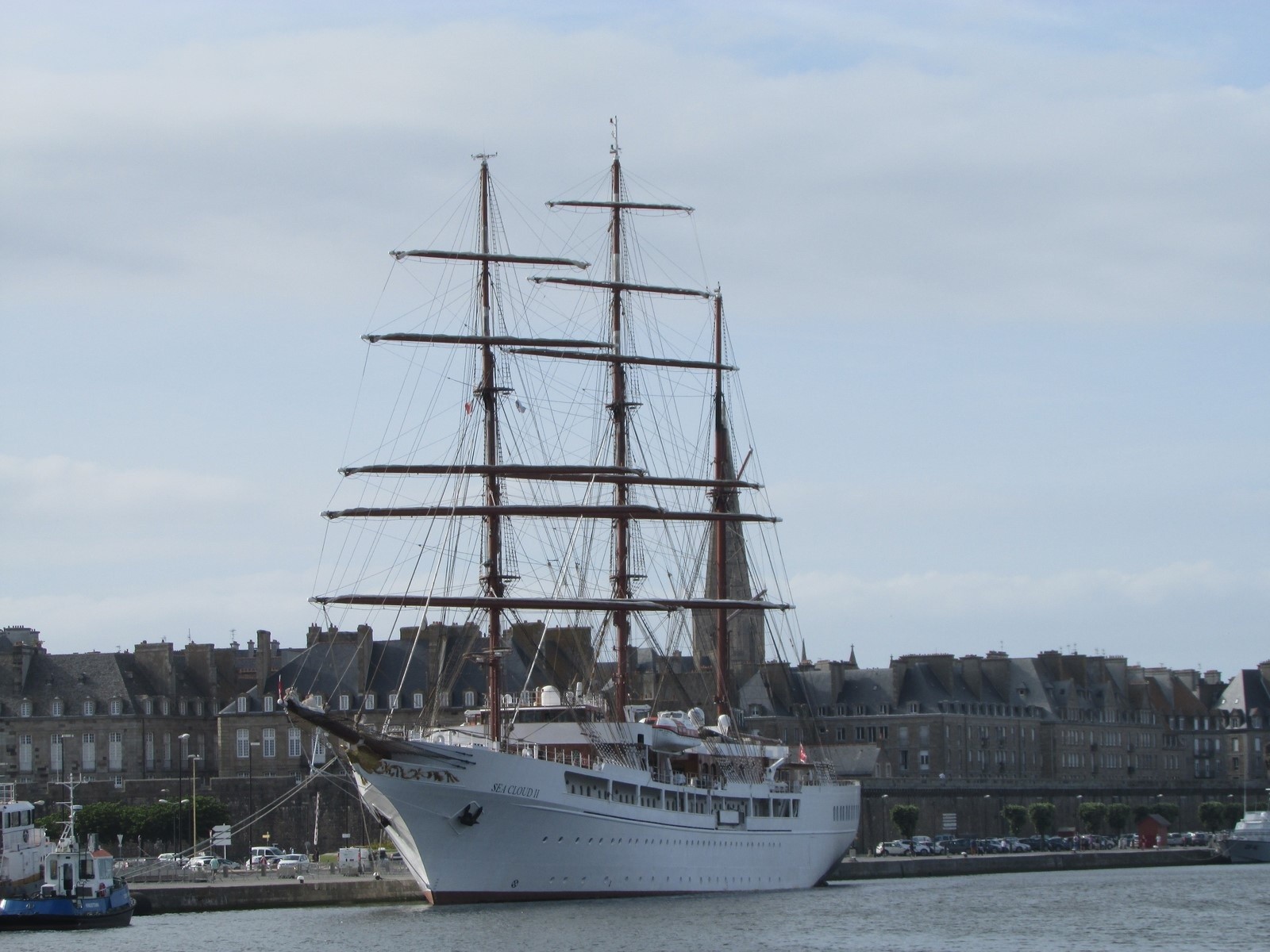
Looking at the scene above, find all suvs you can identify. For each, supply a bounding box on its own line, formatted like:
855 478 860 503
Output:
208 858 241 871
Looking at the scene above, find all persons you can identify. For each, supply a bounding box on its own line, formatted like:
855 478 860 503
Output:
209 856 219 879
312 848 320 862
1072 834 1162 849
970 838 1006 856
260 855 267 864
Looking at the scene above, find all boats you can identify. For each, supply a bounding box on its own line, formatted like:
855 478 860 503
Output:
0 779 138 932
653 710 699 738
1217 787 1270 864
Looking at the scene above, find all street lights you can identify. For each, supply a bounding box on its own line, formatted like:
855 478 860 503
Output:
158 733 204 867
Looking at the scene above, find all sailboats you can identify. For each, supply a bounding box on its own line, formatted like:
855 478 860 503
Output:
276 108 863 905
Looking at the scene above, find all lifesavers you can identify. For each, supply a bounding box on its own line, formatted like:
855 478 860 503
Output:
99 883 107 896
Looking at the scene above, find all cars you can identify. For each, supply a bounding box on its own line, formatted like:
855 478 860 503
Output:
245 846 310 872
156 852 189 866
874 831 1229 858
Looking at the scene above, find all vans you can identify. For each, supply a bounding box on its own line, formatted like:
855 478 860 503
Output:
182 856 217 871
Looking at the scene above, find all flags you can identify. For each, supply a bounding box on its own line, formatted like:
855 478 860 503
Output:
800 743 807 762
